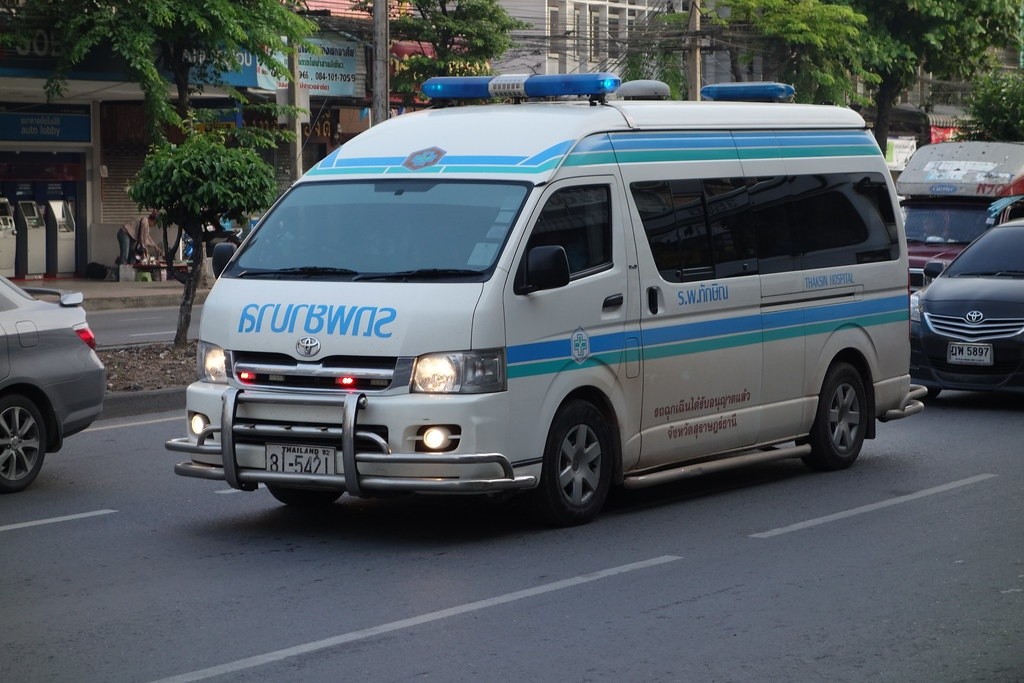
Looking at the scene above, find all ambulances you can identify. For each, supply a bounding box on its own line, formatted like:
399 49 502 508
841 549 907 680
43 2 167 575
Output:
165 74 931 528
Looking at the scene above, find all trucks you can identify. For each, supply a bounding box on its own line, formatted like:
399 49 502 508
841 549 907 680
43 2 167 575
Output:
896 143 1023 294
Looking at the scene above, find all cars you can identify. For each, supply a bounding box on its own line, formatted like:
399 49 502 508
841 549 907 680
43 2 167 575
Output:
908 216 1023 399
1 275 107 492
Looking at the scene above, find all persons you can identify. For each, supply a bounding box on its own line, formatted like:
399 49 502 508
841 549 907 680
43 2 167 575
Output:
919 214 951 243
114 212 162 281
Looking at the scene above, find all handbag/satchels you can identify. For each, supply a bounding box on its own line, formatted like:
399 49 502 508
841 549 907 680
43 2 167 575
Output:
134 242 143 254
